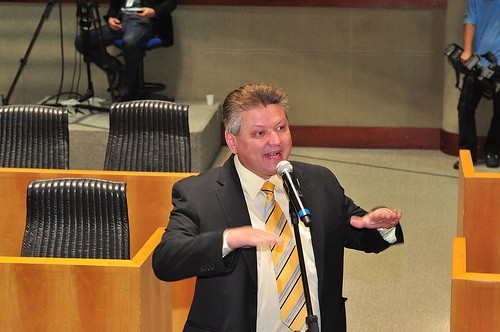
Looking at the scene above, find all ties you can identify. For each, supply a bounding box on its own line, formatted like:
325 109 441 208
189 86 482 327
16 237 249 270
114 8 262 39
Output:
260 182 310 332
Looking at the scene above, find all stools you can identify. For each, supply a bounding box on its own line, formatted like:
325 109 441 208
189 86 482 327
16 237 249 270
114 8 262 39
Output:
112 36 176 103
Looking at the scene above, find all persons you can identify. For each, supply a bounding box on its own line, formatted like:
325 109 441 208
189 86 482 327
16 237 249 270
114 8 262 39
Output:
151 83 404 332
454 0 500 169
74 0 178 103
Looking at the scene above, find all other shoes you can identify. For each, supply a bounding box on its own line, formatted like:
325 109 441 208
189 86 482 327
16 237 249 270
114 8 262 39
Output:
454 155 477 169
118 93 140 101
485 152 498 167
110 64 124 88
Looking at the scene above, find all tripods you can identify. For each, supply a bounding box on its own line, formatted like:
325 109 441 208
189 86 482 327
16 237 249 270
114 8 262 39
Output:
0 0 119 112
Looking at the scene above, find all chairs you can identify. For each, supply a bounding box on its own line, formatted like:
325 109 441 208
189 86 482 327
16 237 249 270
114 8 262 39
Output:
0 103 71 169
102 101 193 173
19 177 131 260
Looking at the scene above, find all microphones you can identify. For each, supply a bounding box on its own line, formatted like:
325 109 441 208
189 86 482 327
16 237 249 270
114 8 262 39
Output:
275 160 312 227
